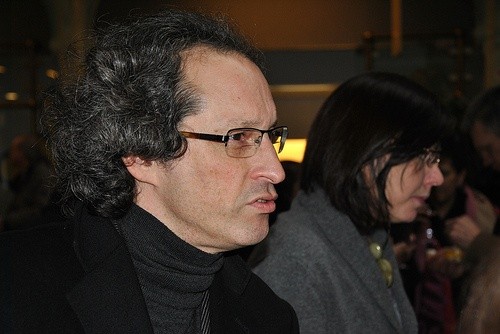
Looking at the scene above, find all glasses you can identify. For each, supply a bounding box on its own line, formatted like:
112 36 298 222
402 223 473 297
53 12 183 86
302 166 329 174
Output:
413 152 441 172
181 121 288 158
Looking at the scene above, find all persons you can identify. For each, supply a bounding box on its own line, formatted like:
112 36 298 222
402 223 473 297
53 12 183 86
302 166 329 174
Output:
1 8 302 334
228 72 500 334
